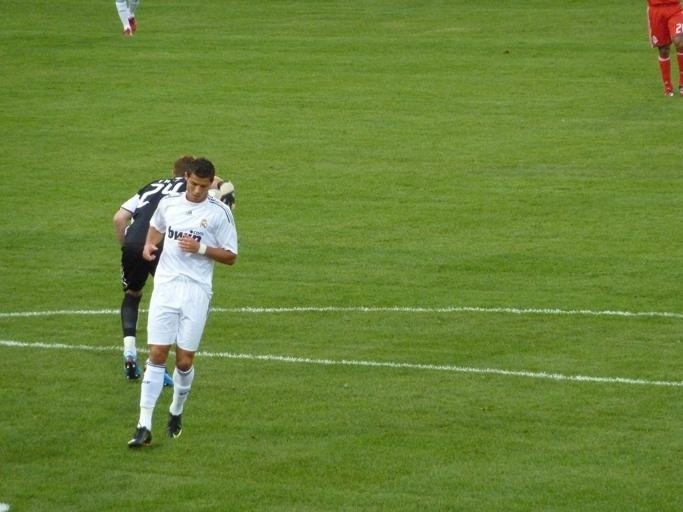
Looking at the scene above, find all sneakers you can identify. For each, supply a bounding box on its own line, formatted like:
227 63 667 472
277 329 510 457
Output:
127 427 153 447
124 351 140 380
166 409 183 440
664 86 683 97
217 181 236 210
144 359 173 386
123 15 138 39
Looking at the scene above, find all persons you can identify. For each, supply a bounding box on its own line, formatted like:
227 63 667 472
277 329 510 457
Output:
114 156 238 448
648 0 683 97
115 0 139 36
